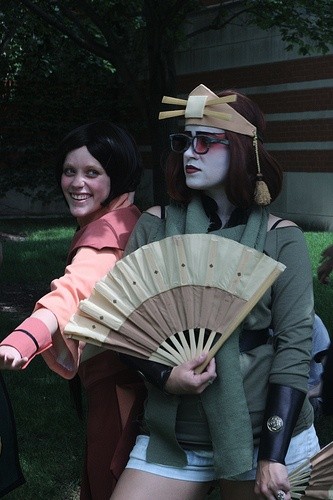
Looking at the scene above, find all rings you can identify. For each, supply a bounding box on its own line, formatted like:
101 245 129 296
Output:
275 490 286 500
208 379 214 385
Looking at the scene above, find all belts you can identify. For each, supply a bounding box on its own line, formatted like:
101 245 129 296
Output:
239 327 274 352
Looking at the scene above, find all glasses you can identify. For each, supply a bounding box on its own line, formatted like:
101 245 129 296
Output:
169 134 229 154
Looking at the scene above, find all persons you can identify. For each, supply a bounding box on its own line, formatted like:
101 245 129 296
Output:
307 313 330 421
0 119 143 500
65 84 333 500
